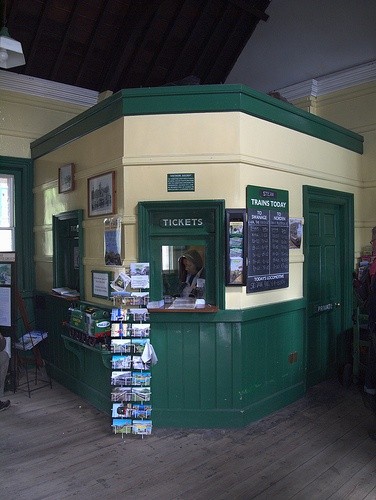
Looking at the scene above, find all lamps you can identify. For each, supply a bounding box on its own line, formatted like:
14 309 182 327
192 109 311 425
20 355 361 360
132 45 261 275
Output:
0 0 26 70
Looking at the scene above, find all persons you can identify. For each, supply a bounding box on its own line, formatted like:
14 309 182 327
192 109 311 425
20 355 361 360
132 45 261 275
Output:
175 249 206 298
0 333 11 412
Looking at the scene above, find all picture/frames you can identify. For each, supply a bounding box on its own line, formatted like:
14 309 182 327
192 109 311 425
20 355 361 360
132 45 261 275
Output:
87 169 116 217
57 162 75 194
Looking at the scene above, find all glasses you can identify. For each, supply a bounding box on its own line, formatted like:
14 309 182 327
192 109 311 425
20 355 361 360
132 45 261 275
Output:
370 239 376 245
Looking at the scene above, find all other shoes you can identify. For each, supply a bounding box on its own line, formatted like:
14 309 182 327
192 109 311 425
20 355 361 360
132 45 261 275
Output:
0 400 11 411
363 384 376 395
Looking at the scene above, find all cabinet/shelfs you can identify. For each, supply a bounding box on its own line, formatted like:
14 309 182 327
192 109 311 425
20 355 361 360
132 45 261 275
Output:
12 289 54 395
351 306 371 385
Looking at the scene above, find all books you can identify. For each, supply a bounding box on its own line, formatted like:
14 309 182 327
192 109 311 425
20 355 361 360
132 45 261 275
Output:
110 263 152 435
13 330 48 351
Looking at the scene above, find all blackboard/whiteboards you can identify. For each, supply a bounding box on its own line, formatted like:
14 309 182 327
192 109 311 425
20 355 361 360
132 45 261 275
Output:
245 184 289 294
